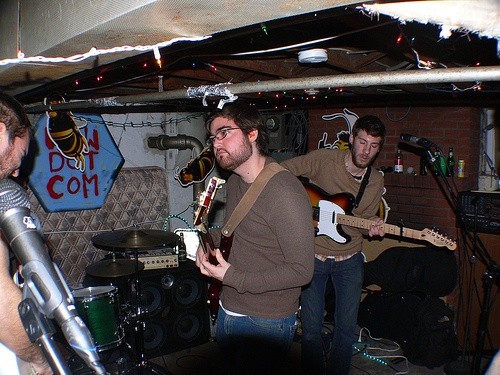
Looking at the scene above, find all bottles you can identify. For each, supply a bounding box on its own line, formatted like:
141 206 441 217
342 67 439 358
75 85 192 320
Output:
446 146 455 177
426 156 437 176
458 159 465 178
393 149 404 174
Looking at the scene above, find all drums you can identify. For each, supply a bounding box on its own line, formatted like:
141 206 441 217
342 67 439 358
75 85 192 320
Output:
71 285 127 354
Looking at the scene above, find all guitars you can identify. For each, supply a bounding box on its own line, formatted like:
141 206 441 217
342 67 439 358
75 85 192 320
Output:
192 176 230 325
301 180 459 252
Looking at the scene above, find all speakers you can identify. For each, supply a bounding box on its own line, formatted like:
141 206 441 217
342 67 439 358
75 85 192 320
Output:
95 251 211 362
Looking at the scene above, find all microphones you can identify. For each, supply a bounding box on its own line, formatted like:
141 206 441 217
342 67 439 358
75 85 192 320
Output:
0 179 108 375
400 132 437 147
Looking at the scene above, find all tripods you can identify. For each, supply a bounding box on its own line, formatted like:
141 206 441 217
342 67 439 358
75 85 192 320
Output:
116 248 170 375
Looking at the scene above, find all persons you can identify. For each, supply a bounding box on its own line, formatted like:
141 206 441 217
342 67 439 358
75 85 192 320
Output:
280 116 385 375
0 92 54 375
196 100 314 343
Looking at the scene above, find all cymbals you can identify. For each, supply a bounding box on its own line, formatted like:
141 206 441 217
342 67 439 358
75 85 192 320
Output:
84 258 145 279
91 227 183 252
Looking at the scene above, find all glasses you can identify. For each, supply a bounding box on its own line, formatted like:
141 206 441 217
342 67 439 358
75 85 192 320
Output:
206 126 250 147
